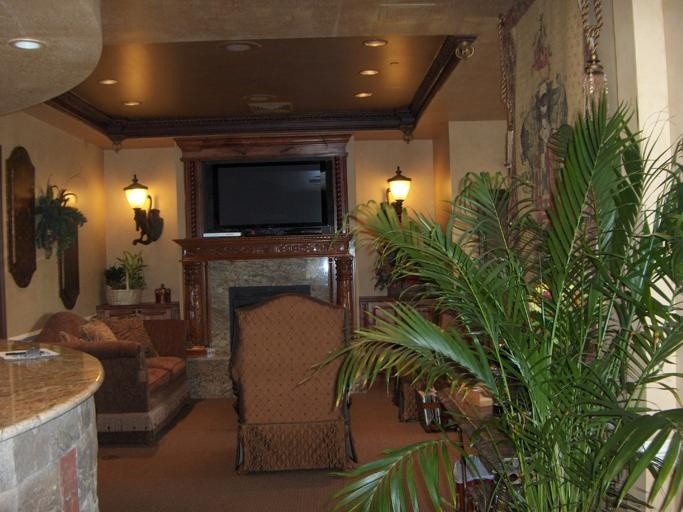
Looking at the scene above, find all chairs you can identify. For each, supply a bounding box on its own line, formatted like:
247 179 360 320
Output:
393 311 489 422
30 310 190 445
228 291 357 475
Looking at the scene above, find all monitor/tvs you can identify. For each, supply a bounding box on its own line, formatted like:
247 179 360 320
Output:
199 157 334 235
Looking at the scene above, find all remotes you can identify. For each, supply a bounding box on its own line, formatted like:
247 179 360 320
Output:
202 232 242 237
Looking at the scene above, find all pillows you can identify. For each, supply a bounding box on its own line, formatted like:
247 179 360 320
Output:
58 313 160 361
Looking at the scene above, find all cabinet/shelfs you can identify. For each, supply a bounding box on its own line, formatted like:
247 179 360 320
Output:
97 301 179 320
360 296 440 372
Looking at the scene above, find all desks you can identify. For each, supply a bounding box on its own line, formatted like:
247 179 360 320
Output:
440 385 537 475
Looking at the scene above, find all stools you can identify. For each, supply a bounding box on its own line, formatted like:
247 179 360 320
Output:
453 454 495 512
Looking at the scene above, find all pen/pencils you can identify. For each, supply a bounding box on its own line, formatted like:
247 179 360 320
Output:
6 351 44 355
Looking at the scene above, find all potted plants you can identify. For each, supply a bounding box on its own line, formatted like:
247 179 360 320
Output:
104 249 148 306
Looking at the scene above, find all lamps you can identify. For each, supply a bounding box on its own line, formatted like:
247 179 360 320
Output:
124 173 163 246
385 165 412 224
